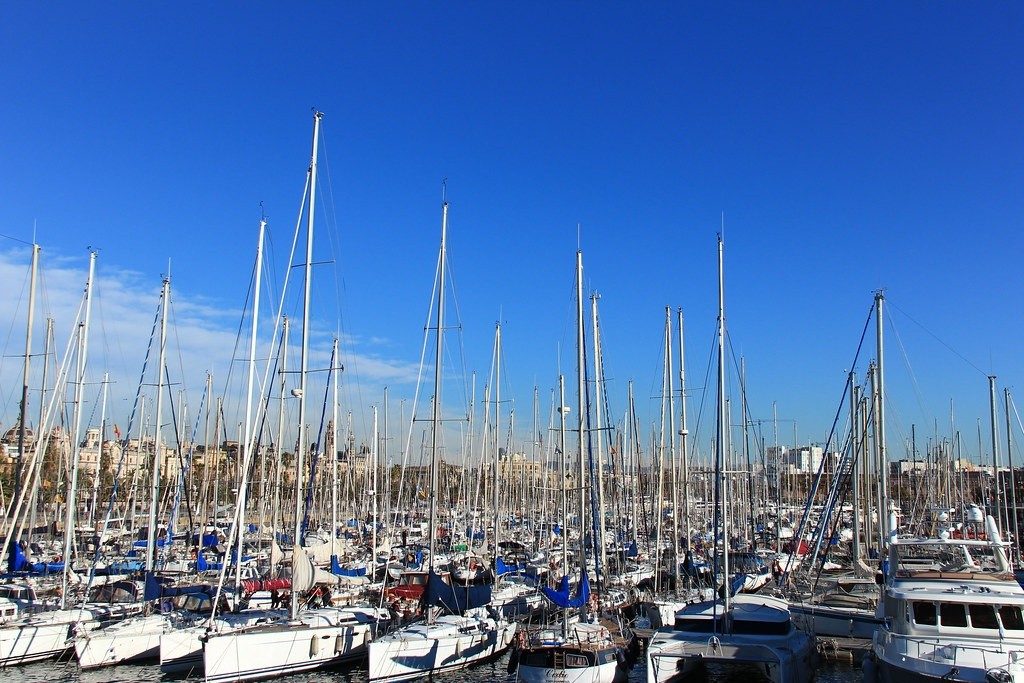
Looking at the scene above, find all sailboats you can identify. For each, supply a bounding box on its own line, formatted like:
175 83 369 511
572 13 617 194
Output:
0 107 1024 683
